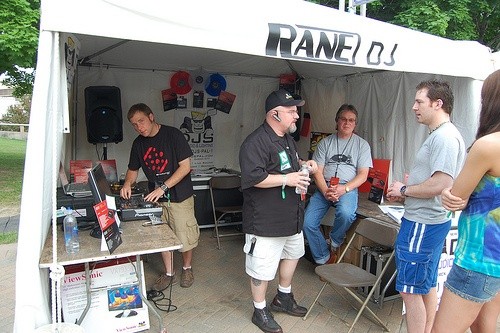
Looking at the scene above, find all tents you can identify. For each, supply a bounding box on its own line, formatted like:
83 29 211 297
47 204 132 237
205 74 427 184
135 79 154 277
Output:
13 0 495 333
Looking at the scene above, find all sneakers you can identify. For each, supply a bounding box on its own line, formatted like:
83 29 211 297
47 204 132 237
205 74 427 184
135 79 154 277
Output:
270 289 307 317
152 273 179 291
251 304 283 333
180 267 194 287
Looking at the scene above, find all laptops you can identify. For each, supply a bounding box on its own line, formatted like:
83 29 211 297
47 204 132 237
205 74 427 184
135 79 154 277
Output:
58 162 92 197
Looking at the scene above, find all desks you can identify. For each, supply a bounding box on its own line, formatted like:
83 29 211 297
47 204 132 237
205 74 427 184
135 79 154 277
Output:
39 217 183 332
307 191 400 226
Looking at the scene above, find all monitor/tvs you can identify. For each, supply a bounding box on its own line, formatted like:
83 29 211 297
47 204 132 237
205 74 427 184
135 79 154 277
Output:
87 163 114 238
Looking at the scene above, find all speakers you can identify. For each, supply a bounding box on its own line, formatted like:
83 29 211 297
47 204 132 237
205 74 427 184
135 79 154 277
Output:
84 86 123 145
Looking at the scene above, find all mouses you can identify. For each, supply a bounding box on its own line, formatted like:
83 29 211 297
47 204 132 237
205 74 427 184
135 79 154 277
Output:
138 202 154 208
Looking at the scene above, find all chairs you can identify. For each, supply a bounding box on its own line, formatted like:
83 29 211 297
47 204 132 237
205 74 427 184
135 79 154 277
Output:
302 218 402 333
209 174 244 250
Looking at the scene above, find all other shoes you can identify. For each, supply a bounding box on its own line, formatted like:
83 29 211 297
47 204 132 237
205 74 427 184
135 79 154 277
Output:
324 252 337 264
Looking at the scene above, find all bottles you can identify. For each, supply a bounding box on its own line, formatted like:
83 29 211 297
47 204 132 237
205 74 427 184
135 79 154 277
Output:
295 164 309 194
63 207 80 255
120 173 125 185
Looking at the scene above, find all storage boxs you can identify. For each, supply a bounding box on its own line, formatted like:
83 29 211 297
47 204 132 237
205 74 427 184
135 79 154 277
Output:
319 225 401 304
191 174 244 228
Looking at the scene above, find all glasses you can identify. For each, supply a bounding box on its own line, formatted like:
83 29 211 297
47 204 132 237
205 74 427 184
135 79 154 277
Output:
338 116 356 123
275 110 299 116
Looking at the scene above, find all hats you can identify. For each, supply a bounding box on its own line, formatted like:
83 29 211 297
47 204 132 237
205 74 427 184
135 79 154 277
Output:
265 89 305 114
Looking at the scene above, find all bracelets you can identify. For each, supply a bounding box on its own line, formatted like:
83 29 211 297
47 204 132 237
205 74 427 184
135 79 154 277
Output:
282 174 286 188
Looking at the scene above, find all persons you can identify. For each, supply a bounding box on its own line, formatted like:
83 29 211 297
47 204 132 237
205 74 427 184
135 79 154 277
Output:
430 69 500 333
238 90 318 333
120 103 200 292
303 103 373 269
387 80 466 333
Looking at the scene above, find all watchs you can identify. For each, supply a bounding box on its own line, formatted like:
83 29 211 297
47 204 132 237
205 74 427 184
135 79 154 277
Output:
160 184 169 192
345 184 350 193
400 185 408 197
